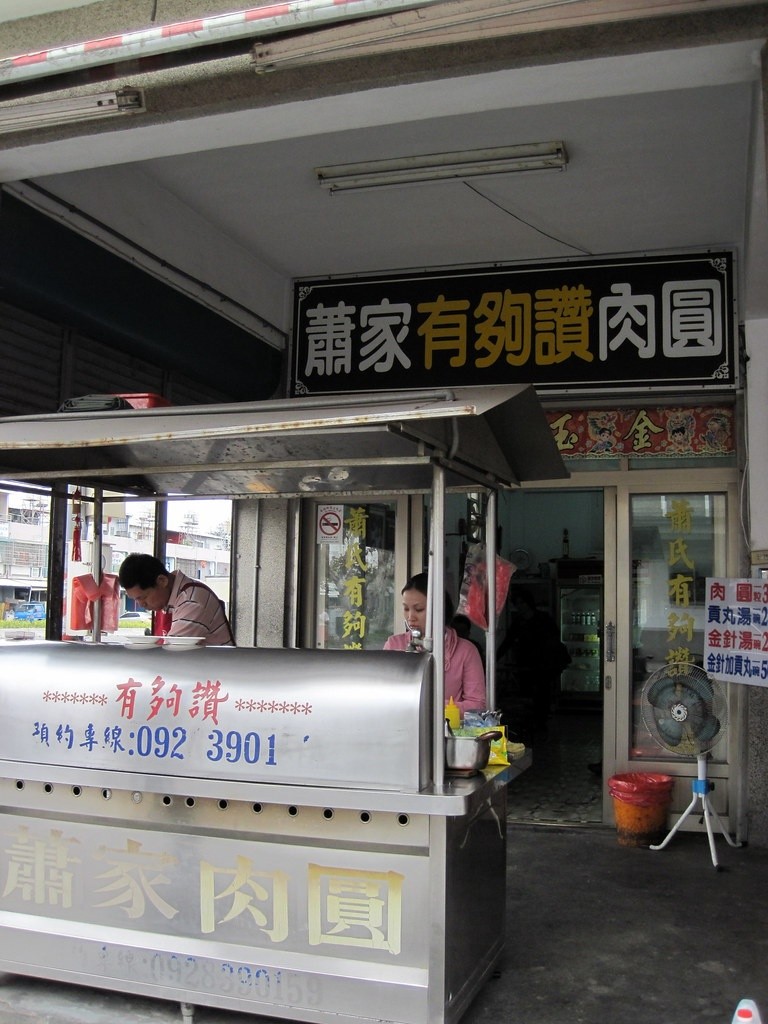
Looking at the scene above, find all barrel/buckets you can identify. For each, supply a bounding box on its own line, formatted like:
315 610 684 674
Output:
538 562 549 578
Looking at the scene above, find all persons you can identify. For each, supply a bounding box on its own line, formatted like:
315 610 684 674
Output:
118 554 234 646
382 571 487 720
498 588 571 745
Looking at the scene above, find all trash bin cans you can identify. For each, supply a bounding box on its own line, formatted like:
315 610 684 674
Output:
607 771 677 849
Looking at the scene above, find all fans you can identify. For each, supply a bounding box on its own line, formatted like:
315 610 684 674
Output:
639 663 748 871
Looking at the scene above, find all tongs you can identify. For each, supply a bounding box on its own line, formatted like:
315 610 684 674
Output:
445 718 455 737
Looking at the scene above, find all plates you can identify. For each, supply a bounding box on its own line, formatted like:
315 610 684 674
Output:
161 636 206 645
125 635 162 644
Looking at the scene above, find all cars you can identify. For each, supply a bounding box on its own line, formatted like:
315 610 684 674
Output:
120 612 152 622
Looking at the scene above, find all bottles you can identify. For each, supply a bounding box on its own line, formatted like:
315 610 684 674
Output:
583 676 600 692
633 598 639 610
632 583 641 596
445 696 460 730
733 1009 758 1024
586 612 591 626
591 613 596 625
576 612 580 626
571 612 576 625
632 645 641 657
571 631 599 642
569 648 598 657
580 613 586 626
633 611 640 626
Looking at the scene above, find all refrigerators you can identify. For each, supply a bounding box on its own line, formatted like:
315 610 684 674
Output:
549 558 648 704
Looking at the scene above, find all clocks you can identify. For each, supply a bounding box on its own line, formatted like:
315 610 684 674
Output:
510 549 531 571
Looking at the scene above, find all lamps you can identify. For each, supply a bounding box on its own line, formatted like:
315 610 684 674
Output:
315 140 568 197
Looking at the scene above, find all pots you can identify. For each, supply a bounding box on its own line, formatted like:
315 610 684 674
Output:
444 731 502 769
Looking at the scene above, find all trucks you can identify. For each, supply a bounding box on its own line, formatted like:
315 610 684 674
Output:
15 601 46 621
